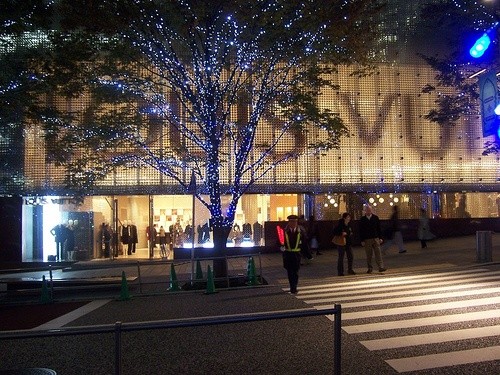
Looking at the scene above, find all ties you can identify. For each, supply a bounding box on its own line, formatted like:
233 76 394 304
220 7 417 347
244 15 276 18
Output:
292 228 294 233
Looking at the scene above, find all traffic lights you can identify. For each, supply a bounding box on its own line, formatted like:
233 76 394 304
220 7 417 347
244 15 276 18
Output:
463 27 495 68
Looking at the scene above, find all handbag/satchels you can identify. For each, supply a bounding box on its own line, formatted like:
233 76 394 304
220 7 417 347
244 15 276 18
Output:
332 234 346 246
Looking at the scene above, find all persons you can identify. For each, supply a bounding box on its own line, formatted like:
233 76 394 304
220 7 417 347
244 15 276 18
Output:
419 208 432 248
185 223 210 244
104 225 111 258
336 213 355 275
280 215 305 295
358 207 386 273
121 220 138 254
298 214 322 258
382 205 407 252
153 223 173 247
50 218 78 261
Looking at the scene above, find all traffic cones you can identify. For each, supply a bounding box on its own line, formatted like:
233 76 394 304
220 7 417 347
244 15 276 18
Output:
164 263 184 292
36 275 59 305
202 264 220 295
244 256 263 286
195 259 205 279
115 270 136 302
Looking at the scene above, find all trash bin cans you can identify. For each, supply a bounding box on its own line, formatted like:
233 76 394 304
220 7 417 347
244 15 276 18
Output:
476 230 493 262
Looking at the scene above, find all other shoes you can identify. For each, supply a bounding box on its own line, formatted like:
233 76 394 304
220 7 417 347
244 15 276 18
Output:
291 287 298 293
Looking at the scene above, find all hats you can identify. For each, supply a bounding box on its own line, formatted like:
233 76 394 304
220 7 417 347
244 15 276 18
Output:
287 215 298 219
